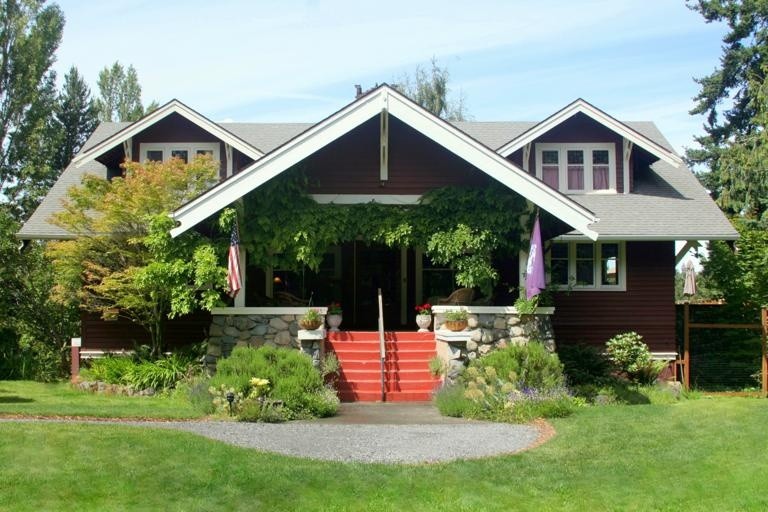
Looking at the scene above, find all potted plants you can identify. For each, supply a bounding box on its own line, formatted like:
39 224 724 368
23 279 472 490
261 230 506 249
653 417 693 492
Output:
444 310 467 333
298 309 322 330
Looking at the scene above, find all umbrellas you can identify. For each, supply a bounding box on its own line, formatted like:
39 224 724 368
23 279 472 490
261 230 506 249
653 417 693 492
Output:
681 258 697 304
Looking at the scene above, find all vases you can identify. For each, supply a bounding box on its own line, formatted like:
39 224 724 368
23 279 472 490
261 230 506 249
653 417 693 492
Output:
326 314 343 332
416 314 432 332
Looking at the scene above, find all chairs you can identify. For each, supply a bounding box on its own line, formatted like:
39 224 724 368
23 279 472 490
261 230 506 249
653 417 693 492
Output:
436 288 476 305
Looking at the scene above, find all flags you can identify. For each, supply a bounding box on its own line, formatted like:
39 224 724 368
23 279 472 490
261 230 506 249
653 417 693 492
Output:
525 215 546 301
225 214 242 300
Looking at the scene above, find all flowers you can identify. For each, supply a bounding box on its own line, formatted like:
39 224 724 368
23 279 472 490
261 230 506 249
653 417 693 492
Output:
415 303 431 315
326 303 342 313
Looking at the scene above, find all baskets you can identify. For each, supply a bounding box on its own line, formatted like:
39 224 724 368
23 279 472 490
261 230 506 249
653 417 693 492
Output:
444 319 468 332
298 319 322 330
